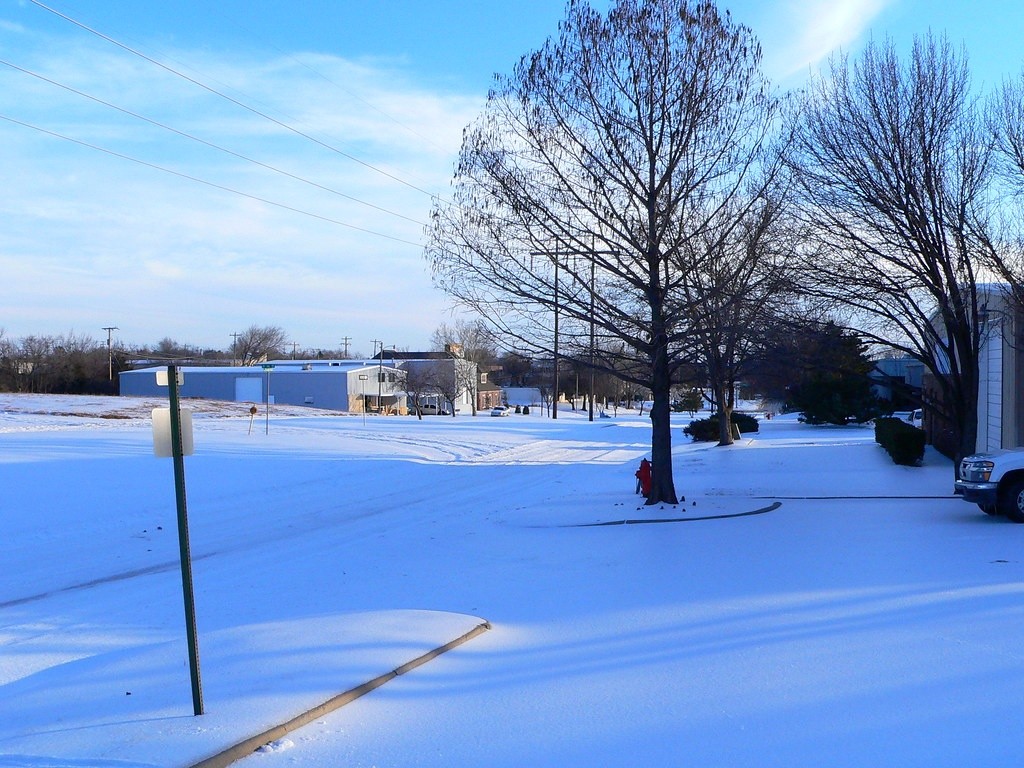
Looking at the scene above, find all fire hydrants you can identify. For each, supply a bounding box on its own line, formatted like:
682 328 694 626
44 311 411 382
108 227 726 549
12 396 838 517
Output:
635 458 652 498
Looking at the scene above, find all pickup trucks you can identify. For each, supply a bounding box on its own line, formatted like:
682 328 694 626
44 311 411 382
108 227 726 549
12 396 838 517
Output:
420 403 451 415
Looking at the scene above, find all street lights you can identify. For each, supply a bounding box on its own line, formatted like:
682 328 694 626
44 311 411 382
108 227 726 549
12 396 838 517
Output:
378 344 396 414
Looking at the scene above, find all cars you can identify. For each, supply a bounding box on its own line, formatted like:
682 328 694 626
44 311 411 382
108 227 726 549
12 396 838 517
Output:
491 406 510 417
908 409 923 430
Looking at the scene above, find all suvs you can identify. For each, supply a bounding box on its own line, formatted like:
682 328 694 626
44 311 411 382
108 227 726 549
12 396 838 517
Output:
954 447 1024 522
